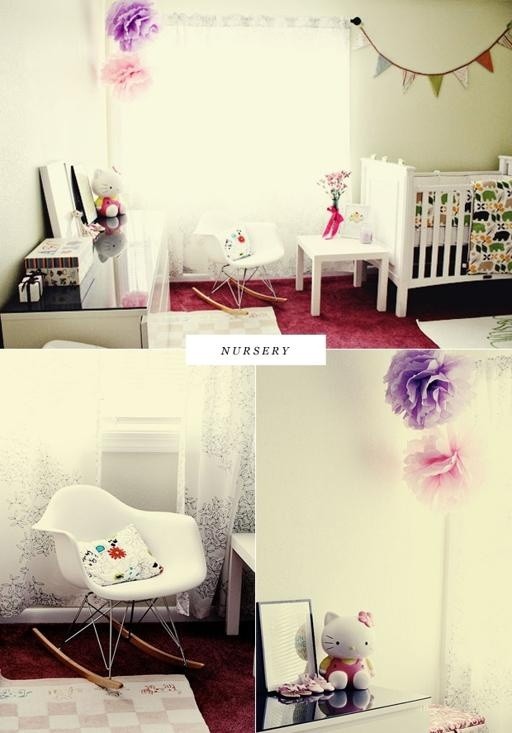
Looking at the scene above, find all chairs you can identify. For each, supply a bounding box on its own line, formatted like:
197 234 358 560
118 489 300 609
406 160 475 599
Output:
191 214 289 316
31 482 209 691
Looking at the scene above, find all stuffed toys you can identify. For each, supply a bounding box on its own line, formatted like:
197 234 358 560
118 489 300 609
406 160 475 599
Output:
318 690 375 717
91 166 128 217
318 610 377 690
93 217 128 263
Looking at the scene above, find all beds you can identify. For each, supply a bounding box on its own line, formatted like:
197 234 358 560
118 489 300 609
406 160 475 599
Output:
361 153 511 317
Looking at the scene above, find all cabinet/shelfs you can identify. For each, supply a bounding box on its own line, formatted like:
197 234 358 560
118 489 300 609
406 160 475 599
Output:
1 216 171 347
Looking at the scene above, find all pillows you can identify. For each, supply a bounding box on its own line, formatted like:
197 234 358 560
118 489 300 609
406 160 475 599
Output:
76 521 164 587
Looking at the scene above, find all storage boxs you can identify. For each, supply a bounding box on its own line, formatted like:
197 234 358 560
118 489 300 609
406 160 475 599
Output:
18 238 94 301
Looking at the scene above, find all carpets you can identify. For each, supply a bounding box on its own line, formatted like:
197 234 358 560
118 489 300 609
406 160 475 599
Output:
415 315 512 348
2 674 211 733
169 306 282 347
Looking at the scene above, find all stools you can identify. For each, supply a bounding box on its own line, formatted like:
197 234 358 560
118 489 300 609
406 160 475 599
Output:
225 531 255 635
294 233 388 317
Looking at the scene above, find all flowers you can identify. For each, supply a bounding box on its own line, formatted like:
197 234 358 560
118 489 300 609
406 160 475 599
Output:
383 348 478 505
315 170 354 200
100 0 163 100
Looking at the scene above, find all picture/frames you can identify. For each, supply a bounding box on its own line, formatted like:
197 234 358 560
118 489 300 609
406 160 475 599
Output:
255 597 318 693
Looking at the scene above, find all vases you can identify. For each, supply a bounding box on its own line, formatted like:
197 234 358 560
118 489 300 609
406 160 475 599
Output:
323 206 343 239
